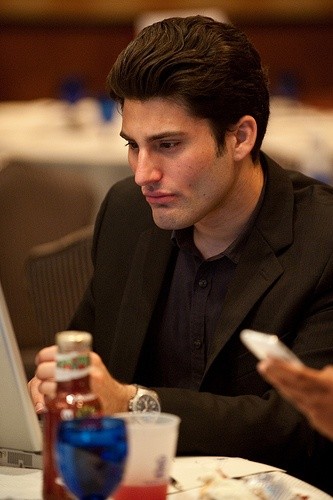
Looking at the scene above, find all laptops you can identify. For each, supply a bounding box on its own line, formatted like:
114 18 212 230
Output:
1 287 44 469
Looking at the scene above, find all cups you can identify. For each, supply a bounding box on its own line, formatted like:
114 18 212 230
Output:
57 418 128 500
114 412 181 500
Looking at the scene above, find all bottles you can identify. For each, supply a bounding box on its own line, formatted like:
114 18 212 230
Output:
41 331 104 500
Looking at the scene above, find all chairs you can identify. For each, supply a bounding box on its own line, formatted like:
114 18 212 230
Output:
0 166 104 386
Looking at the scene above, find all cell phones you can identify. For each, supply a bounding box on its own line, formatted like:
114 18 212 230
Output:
239 329 306 367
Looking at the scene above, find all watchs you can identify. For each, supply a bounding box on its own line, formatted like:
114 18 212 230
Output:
127 383 160 424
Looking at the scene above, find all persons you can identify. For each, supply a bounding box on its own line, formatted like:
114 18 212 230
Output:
253 355 331 442
24 13 332 498
